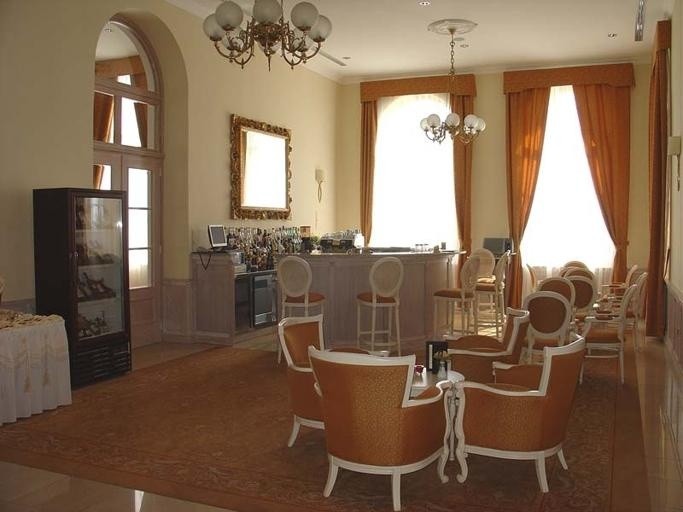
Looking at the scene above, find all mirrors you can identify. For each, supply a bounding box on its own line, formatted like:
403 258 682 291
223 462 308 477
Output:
230 113 292 221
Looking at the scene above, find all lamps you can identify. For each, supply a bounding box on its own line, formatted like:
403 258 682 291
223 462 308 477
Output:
420 18 486 146
203 0 333 71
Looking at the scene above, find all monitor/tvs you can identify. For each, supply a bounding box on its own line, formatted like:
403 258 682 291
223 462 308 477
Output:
207 225 228 252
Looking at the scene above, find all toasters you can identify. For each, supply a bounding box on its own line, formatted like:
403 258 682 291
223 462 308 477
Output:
483 237 514 254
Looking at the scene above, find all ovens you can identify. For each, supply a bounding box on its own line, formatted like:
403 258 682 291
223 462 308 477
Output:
234 270 278 334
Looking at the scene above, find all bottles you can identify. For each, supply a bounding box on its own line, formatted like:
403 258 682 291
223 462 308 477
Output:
79 314 100 335
437 362 446 378
79 279 93 298
83 272 104 298
228 225 300 270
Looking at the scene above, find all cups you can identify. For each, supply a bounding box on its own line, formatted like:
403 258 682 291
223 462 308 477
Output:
421 244 425 251
414 365 424 377
425 244 429 252
415 244 421 252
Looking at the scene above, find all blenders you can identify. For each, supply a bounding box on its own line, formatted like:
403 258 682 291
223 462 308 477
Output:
300 226 312 254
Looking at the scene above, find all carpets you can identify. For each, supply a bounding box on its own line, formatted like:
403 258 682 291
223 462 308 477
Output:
0 346 650 512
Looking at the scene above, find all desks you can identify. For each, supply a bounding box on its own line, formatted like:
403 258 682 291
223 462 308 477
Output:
1 309 72 425
